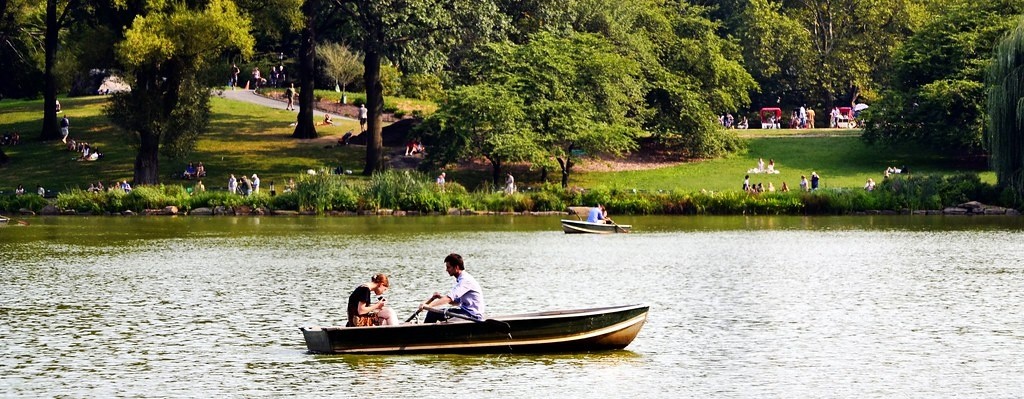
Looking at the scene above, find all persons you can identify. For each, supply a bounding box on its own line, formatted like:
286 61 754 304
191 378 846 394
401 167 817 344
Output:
231 62 299 111
405 138 427 159
790 103 815 129
716 112 748 129
437 172 445 192
811 172 819 190
587 202 612 225
743 157 779 193
12 180 132 198
284 103 367 193
0 100 101 162
346 253 483 327
184 161 260 196
884 166 909 179
506 172 514 193
799 175 808 190
865 177 875 192
830 106 866 128
782 182 788 191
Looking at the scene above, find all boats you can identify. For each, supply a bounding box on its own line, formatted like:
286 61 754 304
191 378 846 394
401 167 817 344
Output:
298 304 649 354
560 219 632 234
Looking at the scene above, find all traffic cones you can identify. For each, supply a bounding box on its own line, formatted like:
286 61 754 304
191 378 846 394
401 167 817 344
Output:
244 80 250 90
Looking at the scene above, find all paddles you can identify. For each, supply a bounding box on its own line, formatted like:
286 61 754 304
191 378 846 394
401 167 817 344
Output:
405 295 437 322
574 210 582 220
423 305 510 336
605 215 627 232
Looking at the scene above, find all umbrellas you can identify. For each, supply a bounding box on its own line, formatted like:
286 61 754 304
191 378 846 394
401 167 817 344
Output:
852 103 868 111
